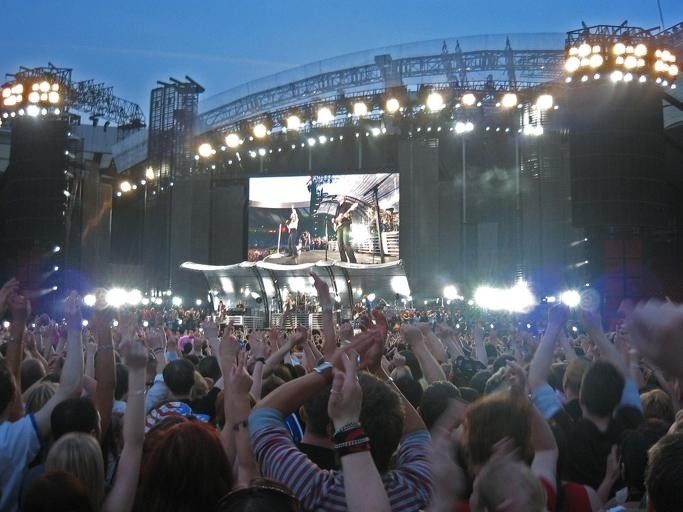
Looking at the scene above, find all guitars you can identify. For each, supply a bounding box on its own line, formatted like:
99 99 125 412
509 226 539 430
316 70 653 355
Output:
333 203 359 232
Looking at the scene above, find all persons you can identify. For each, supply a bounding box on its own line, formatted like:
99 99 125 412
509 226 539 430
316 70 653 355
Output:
0 271 683 512
247 201 399 265
